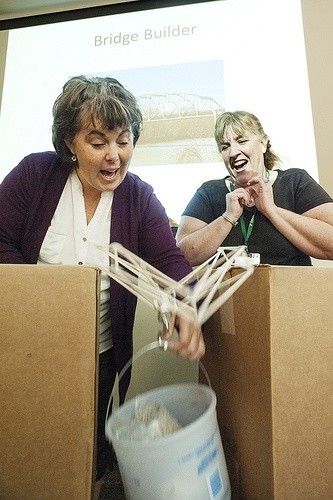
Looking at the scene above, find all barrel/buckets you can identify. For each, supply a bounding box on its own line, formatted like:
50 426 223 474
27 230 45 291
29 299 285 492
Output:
104 340 232 500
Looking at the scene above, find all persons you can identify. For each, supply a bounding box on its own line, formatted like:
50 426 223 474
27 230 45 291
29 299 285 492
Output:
0 76 205 484
175 112 332 266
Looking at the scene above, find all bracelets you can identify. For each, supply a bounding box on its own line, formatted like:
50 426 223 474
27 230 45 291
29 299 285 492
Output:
222 212 238 226
